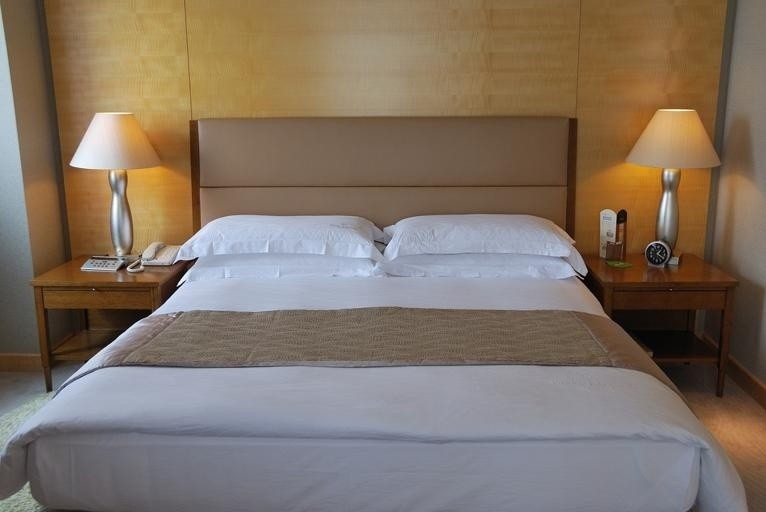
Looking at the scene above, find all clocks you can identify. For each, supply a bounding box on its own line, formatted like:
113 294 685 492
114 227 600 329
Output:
644 241 671 267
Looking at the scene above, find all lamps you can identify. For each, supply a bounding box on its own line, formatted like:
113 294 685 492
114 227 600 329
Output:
69 112 161 261
626 109 723 256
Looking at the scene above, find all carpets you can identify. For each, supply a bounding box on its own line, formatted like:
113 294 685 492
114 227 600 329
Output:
0 359 87 512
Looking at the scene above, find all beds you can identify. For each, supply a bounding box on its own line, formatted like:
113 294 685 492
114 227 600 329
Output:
0 117 749 512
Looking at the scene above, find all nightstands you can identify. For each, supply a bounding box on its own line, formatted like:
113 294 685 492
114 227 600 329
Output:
582 253 738 399
28 255 194 393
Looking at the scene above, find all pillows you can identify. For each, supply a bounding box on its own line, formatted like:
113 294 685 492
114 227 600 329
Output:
379 255 589 281
385 214 575 256
175 215 385 260
176 256 383 285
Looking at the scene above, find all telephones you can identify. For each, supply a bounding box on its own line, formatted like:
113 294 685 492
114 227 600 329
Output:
126 242 182 272
